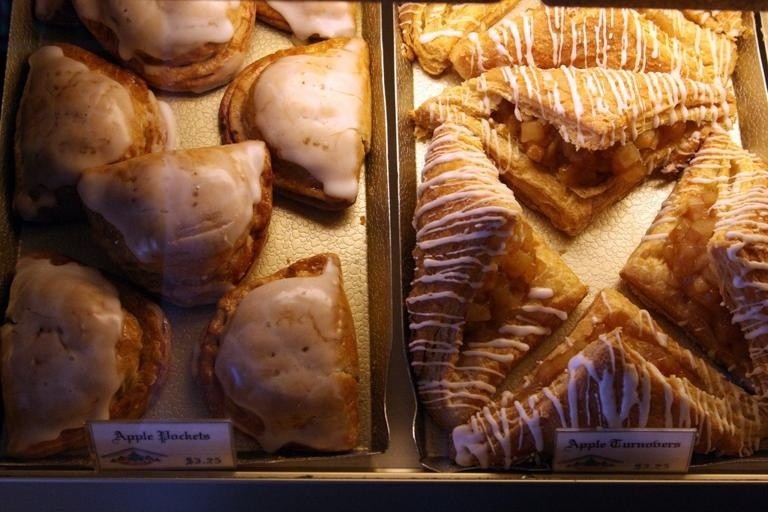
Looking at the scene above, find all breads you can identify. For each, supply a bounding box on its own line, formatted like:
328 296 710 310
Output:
257 0 358 37
74 0 257 96
613 130 767 398
77 141 275 309
395 3 746 80
0 242 172 457
192 251 360 452
216 32 373 215
13 40 178 222
407 68 738 238
450 288 766 468
407 120 590 429
